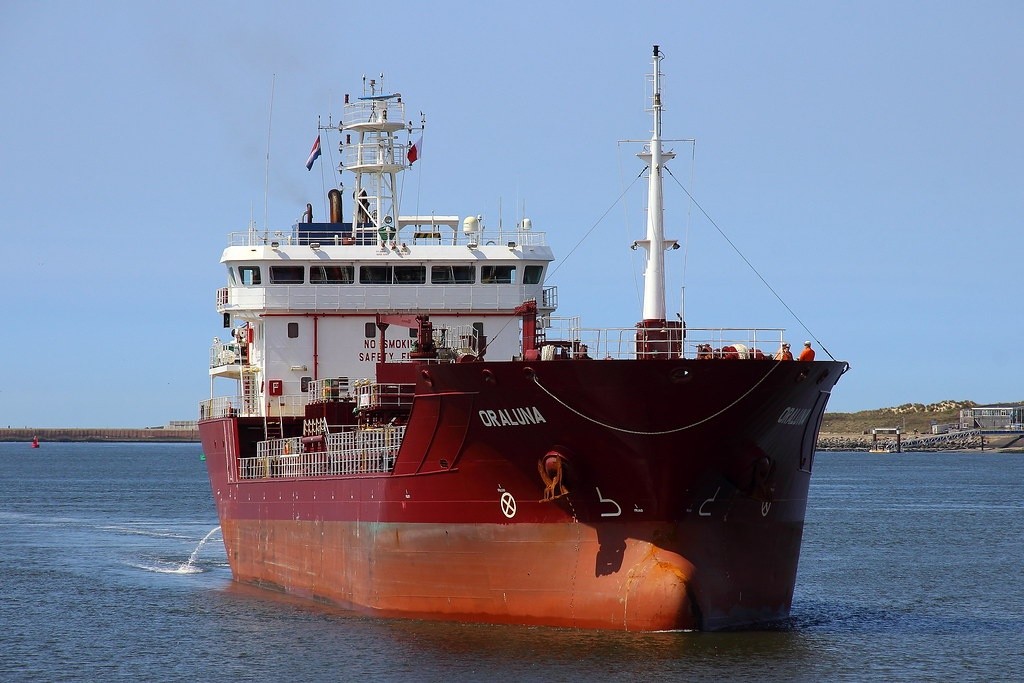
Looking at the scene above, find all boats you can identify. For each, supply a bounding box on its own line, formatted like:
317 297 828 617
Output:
194 41 852 636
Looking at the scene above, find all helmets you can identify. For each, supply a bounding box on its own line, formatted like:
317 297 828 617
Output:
804 341 811 346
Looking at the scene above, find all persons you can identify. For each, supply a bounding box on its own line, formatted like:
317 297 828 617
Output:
776 341 815 361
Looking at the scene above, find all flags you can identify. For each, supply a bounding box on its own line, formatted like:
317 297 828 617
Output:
406 135 423 164
304 133 322 172
